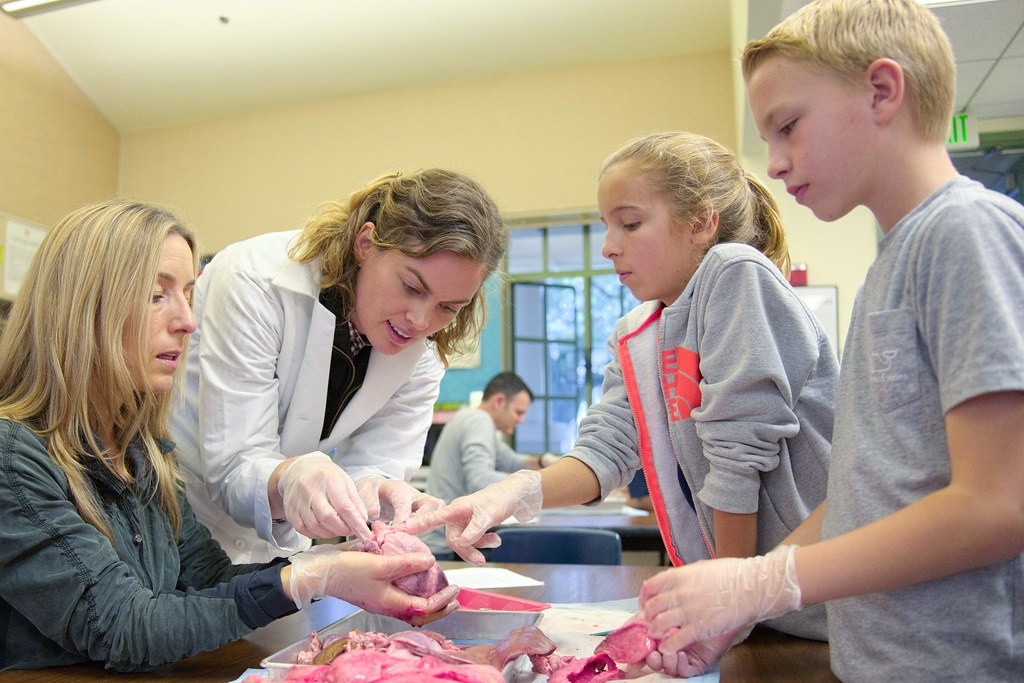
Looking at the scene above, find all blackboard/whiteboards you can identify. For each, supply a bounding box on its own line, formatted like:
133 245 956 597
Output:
792 284 840 365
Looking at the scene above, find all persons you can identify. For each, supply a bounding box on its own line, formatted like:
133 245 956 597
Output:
640 0 1024 683
160 165 510 564
426 371 563 561
0 197 461 676
393 131 842 641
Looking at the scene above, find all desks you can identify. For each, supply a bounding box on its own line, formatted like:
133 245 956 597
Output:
0 489 842 683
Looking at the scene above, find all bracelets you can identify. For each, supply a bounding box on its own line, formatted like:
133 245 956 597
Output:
537 452 545 468
